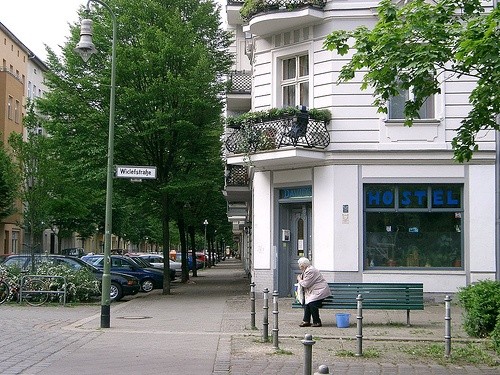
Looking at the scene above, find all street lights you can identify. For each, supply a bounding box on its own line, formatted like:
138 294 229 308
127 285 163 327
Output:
74 0 117 328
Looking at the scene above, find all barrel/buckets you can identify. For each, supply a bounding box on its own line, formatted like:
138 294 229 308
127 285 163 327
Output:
335 313 351 328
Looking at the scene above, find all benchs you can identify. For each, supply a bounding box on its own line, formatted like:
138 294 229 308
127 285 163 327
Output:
292 283 424 327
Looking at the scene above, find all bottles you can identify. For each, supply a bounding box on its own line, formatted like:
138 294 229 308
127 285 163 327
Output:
370 260 374 267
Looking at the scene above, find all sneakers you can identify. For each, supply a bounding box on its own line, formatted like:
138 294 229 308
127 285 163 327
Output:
299 321 310 327
312 323 321 326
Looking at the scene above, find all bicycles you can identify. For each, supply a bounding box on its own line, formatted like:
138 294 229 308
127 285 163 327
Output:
0 266 49 307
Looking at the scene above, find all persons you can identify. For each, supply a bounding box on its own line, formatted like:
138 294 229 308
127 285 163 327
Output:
297 256 332 327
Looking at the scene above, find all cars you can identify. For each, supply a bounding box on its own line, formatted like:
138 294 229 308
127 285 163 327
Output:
127 250 224 278
0 253 141 302
74 254 163 293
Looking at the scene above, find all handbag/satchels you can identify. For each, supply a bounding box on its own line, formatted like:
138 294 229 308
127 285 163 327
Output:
297 274 304 305
